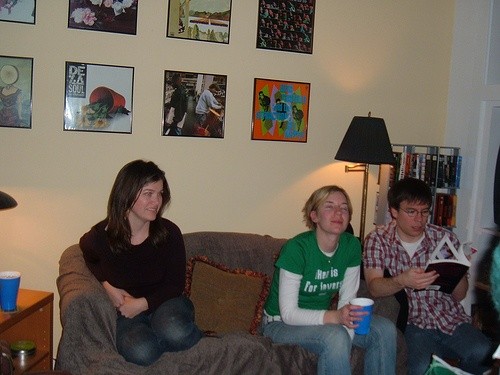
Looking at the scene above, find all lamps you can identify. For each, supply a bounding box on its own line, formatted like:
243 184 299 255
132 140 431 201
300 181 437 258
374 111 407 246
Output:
334 111 397 246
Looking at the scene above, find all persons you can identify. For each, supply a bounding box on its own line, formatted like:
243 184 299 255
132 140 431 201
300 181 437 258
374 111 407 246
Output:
168 74 224 135
262 185 396 375
490 243 500 311
78 160 202 364
364 178 491 375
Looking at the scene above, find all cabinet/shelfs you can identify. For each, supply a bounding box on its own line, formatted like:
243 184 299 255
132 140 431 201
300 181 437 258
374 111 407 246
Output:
0 289 54 375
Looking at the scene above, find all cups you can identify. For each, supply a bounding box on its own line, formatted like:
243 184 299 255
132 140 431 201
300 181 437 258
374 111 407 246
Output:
0 271 22 312
349 297 374 334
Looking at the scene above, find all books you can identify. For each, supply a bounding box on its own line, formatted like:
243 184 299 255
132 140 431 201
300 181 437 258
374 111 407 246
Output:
374 152 462 230
413 234 478 294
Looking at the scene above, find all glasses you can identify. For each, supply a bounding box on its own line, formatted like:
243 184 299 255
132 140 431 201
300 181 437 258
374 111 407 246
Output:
399 207 430 217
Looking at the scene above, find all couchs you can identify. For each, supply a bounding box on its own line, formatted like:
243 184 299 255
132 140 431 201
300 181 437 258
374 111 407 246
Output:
53 231 405 375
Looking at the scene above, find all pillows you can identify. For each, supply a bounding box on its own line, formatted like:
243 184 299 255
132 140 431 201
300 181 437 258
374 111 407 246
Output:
187 260 271 337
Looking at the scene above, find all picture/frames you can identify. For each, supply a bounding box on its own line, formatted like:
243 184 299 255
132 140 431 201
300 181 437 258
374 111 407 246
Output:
162 70 227 139
68 0 138 35
0 0 37 25
256 0 316 54
166 0 232 44
0 55 34 129
63 61 135 134
251 77 311 143
